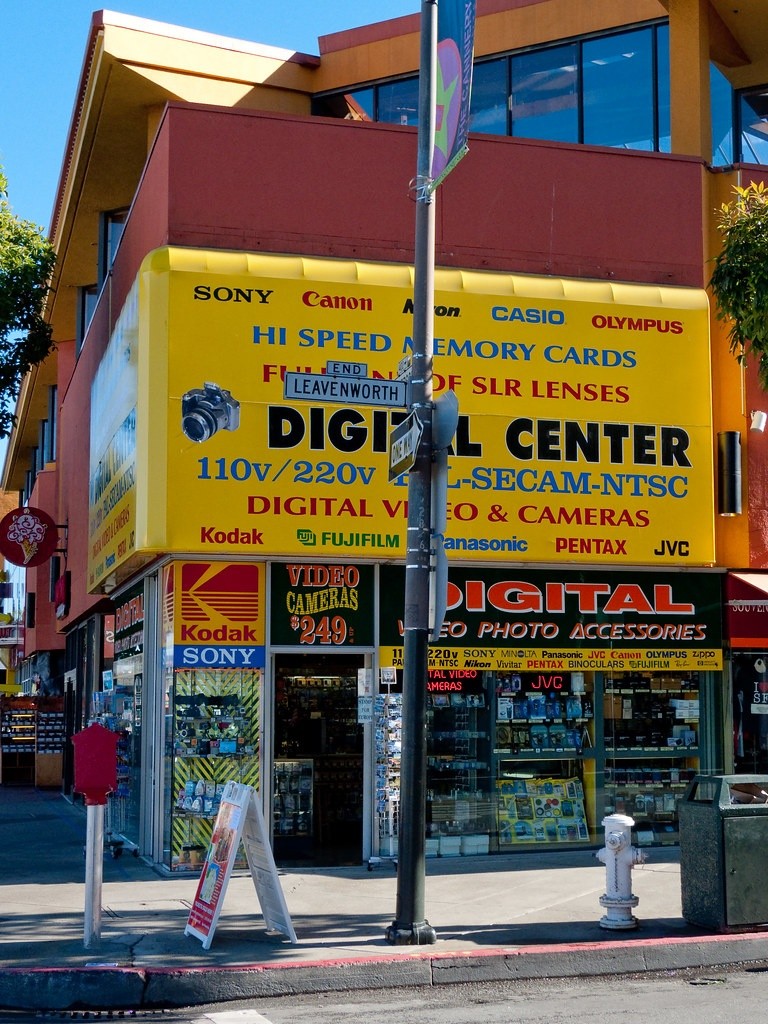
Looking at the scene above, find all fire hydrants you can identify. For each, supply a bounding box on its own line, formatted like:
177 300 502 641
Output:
596 814 649 929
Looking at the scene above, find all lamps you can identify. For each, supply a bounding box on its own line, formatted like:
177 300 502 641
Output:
717 431 743 517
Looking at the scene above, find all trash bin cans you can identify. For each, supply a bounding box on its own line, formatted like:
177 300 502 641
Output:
677 774 768 934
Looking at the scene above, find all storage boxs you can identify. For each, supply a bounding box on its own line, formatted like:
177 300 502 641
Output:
667 738 684 746
680 729 695 746
603 693 612 719
661 678 671 690
460 836 479 856
437 835 459 856
671 678 681 690
612 696 622 719
474 834 490 854
650 678 660 689
623 700 632 708
425 839 440 857
667 699 699 721
622 709 632 720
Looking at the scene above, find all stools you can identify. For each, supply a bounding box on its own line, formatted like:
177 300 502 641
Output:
313 782 331 843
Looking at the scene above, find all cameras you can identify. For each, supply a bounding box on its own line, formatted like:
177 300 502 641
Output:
216 737 244 753
177 728 196 736
181 383 240 443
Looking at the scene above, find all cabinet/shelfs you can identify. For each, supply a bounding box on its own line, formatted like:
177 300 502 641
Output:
0 696 65 787
274 758 313 837
